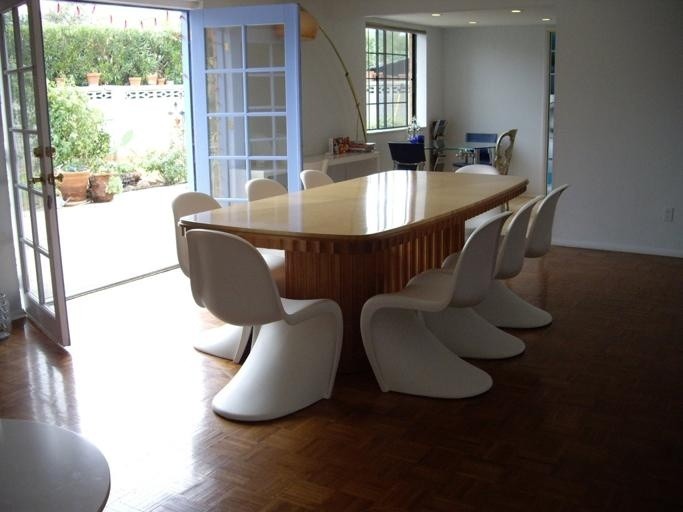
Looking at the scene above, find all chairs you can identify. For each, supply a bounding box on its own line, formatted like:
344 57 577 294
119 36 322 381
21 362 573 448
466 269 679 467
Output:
359 212 513 398
405 194 544 359
442 183 569 329
245 179 287 202
454 164 504 242
184 228 342 423
172 190 287 362
300 169 333 191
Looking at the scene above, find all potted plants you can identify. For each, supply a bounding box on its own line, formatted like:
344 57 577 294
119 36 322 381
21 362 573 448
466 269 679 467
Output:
5 54 123 207
123 48 175 87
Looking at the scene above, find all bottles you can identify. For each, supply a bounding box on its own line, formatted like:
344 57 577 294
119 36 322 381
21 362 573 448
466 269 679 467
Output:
337 136 343 154
407 120 419 144
416 127 424 144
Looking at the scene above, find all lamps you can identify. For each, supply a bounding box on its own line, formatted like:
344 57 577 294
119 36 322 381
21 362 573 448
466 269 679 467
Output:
272 0 375 150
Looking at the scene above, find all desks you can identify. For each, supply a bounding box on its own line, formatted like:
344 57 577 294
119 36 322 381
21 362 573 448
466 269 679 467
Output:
0 414 113 511
178 168 528 379
